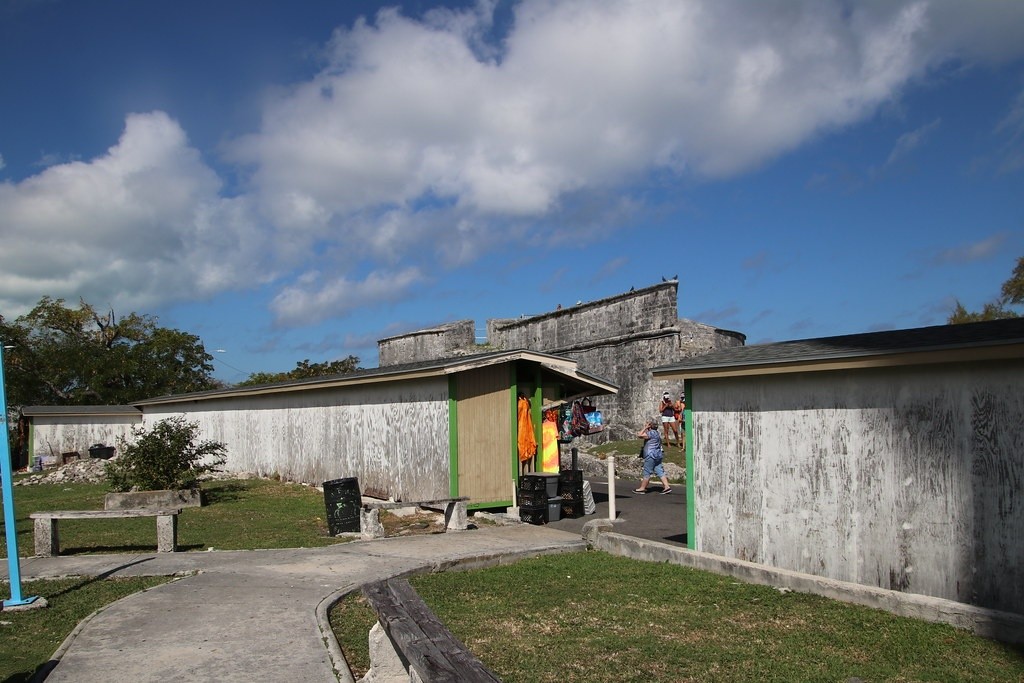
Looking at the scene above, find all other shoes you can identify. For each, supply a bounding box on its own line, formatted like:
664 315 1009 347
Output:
678 448 686 453
632 489 645 495
659 487 671 495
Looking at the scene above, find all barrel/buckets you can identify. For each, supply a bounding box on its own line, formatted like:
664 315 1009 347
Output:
88 446 115 460
321 477 363 536
41 456 58 470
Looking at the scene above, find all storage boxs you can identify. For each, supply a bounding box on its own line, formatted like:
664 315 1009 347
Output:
518 469 595 525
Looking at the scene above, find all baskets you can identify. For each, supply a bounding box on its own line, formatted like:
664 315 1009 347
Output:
557 471 585 519
518 474 549 526
583 480 596 515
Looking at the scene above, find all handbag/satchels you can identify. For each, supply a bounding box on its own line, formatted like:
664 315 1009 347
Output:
674 407 678 420
559 397 603 443
639 446 645 459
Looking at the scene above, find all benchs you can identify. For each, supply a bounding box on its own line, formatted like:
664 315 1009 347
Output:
362 579 505 683
30 509 182 557
360 496 472 537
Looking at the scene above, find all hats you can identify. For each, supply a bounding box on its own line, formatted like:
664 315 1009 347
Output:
682 399 685 402
681 393 685 397
664 394 669 398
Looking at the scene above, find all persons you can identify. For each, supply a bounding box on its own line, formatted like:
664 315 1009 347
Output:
659 391 686 452
631 419 673 495
